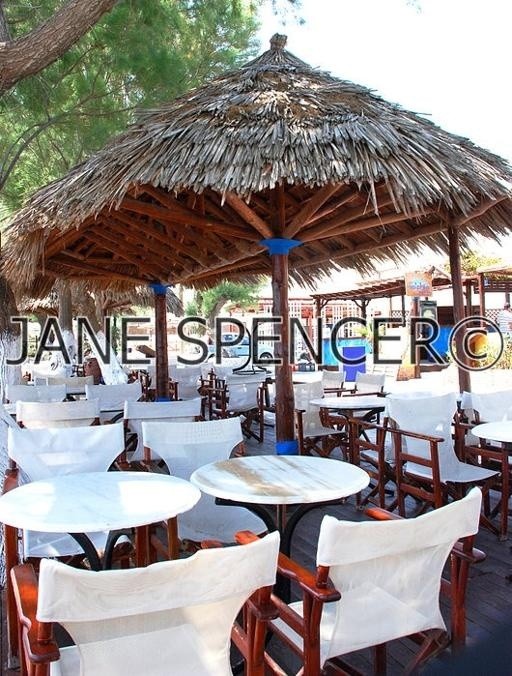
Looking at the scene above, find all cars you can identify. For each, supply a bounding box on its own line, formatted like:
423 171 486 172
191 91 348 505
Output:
205 332 275 366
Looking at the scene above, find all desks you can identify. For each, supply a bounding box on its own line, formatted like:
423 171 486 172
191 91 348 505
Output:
470 421 512 519
0 470 202 572
291 371 324 383
190 451 371 674
309 396 385 464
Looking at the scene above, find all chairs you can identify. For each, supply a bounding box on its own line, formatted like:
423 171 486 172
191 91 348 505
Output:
292 380 349 461
4 382 69 405
461 388 512 517
6 421 147 657
84 380 143 413
211 365 234 418
232 485 488 676
46 375 95 390
205 371 267 444
9 396 101 469
167 366 212 421
113 396 207 471
384 393 512 542
139 416 288 566
325 370 386 443
321 369 347 397
141 364 172 402
10 529 281 676
349 393 432 509
